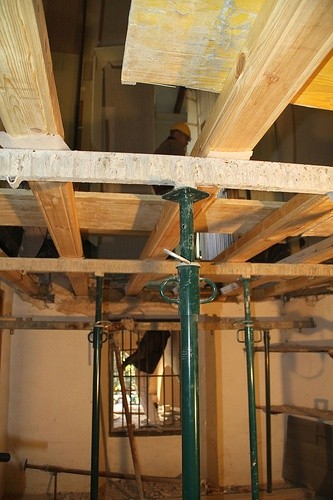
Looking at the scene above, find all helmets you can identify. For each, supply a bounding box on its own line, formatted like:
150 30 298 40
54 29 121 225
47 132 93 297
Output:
170 122 190 137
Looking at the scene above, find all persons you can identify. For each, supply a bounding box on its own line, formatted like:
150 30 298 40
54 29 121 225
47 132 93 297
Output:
151 122 192 194
